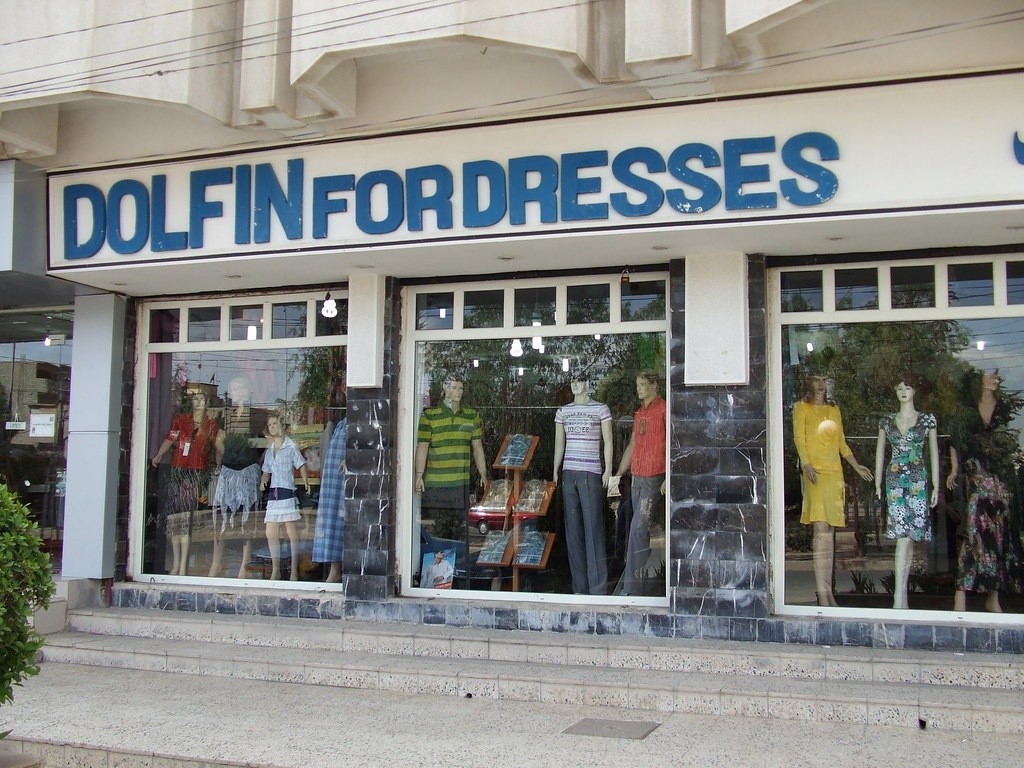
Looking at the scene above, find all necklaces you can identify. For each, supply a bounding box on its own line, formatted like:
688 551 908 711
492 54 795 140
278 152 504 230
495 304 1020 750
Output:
194 421 202 425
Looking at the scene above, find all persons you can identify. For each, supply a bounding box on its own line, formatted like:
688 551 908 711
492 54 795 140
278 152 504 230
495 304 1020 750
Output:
790 368 872 608
260 417 311 581
152 392 219 576
945 360 1023 614
415 372 488 590
876 374 940 610
310 413 347 584
208 377 269 578
553 369 613 595
616 369 666 595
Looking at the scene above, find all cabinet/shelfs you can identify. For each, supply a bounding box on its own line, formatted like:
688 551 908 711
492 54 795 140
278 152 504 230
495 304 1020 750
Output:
475 434 556 592
